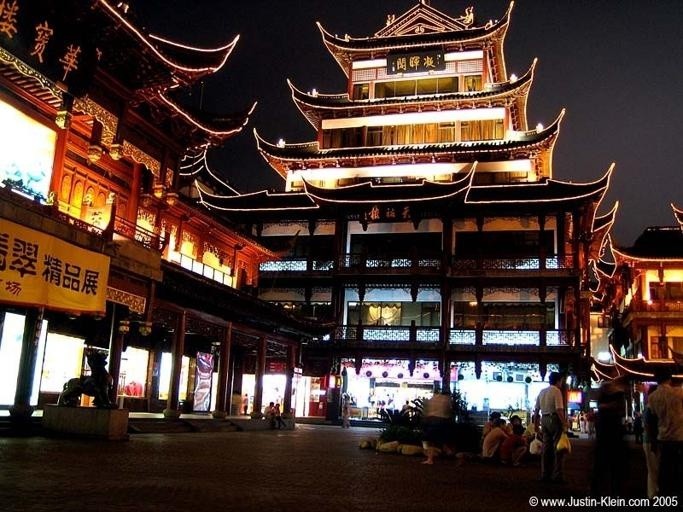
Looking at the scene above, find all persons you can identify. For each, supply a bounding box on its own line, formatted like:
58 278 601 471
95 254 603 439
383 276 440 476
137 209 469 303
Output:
341 370 682 504
243 394 248 415
264 402 276 429
275 404 287 430
